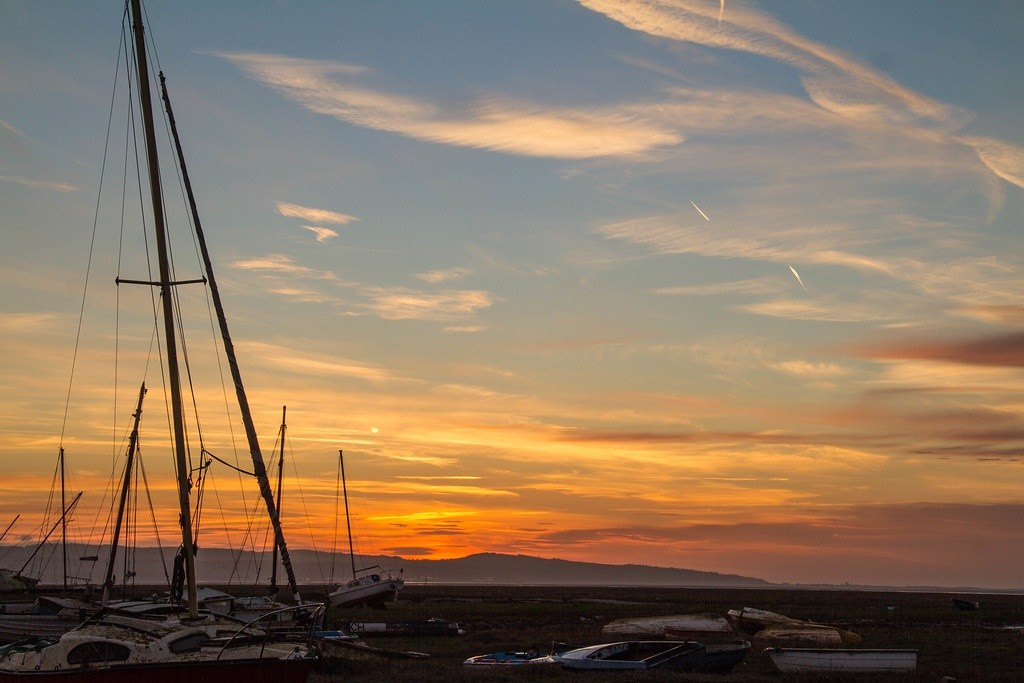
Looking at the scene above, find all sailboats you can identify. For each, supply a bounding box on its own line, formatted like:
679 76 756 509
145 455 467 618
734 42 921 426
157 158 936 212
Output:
0 444 100 642
324 450 404 608
233 405 327 623
0 1 319 683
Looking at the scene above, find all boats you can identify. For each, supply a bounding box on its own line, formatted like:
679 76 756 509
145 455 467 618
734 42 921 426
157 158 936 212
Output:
761 647 920 675
462 651 558 667
552 641 706 672
602 613 733 640
727 607 802 630
753 623 863 650
705 639 751 671
